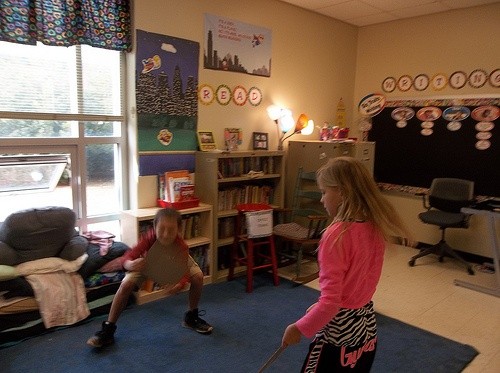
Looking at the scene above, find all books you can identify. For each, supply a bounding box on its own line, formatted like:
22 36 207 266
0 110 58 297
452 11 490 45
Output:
157 169 195 202
138 216 210 293
217 157 273 239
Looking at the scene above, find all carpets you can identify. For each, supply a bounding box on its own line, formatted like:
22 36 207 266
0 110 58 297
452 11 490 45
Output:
0 271 479 373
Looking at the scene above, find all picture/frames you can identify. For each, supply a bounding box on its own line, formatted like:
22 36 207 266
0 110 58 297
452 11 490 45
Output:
197 130 218 152
253 132 268 151
224 128 243 150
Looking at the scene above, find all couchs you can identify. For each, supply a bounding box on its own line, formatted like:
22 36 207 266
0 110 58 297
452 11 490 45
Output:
0 207 135 345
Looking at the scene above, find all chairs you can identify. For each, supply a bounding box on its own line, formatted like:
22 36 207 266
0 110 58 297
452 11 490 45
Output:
273 167 330 285
408 178 475 276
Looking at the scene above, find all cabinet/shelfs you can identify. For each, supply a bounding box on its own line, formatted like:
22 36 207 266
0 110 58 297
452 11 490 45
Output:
121 202 213 306
285 139 376 229
195 151 284 283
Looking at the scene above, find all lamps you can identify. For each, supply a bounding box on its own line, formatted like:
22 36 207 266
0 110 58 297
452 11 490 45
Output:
267 105 314 150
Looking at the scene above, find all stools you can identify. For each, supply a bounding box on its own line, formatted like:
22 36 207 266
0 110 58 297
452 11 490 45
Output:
227 203 279 292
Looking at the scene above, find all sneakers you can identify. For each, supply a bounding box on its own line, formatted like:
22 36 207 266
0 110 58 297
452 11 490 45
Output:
182 308 212 332
87 321 117 347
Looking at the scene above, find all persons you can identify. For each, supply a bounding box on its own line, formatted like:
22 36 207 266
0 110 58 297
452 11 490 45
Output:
281 157 417 373
86 207 213 348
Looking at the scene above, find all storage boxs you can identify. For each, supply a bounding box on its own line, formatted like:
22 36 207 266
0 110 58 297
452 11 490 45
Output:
156 197 200 210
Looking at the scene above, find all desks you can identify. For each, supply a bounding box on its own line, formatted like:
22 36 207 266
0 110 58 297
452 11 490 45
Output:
453 200 500 298
